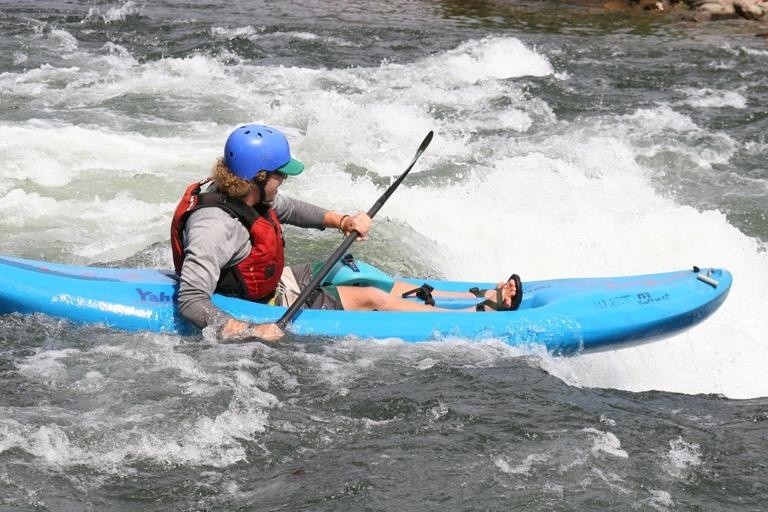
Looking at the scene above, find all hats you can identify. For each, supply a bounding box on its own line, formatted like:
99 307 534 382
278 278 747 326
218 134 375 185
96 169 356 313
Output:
280 158 305 175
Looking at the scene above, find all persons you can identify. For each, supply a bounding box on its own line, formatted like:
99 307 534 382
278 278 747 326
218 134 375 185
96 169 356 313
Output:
169 123 524 341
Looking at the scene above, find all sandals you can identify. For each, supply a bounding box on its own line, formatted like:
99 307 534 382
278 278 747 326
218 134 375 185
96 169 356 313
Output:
471 274 522 312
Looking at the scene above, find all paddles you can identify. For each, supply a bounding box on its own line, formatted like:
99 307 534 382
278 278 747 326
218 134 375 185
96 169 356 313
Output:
277 129 434 332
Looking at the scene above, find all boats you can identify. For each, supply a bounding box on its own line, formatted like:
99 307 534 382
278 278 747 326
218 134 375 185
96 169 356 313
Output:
0 255 732 359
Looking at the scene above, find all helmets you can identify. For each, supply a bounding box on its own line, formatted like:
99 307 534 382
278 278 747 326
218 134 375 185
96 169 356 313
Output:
224 124 290 180
